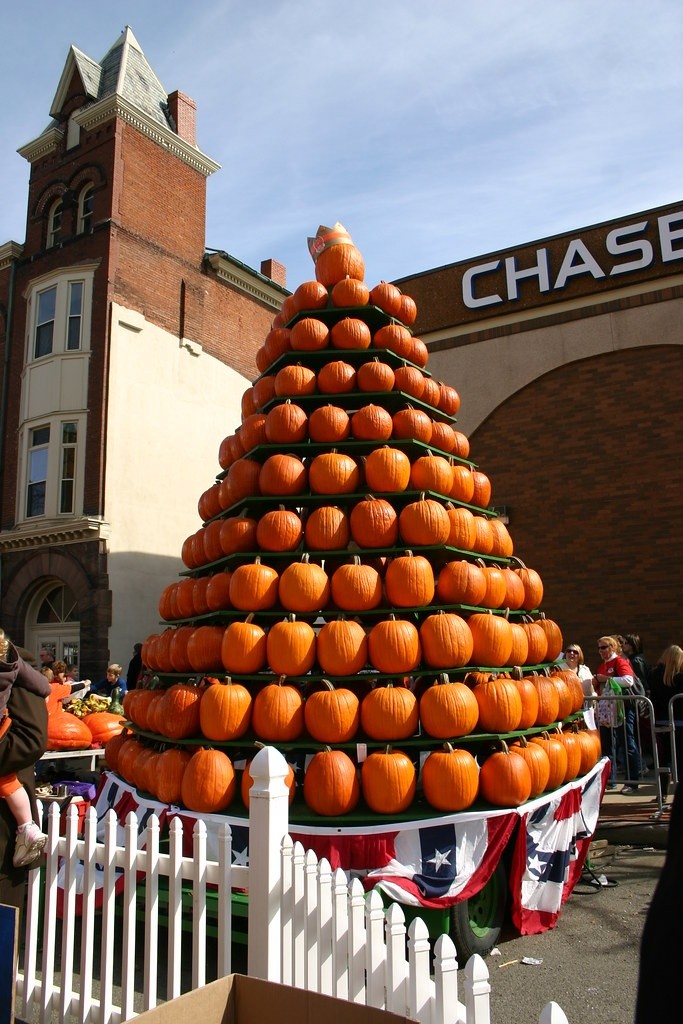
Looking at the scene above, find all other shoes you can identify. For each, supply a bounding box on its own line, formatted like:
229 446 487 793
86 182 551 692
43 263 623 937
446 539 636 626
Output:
656 794 668 802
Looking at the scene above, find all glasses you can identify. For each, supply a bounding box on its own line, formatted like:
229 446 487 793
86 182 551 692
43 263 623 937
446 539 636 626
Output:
40 654 48 657
567 649 578 654
598 645 610 650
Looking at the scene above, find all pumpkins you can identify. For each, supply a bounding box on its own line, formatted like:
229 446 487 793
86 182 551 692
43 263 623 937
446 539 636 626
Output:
46 243 608 819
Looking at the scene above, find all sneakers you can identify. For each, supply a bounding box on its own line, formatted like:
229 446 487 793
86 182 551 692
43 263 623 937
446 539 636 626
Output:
620 785 638 795
603 785 618 791
13 821 47 867
638 767 648 775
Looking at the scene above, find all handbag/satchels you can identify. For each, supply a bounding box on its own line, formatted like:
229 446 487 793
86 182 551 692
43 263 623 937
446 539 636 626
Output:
596 679 617 728
605 677 625 726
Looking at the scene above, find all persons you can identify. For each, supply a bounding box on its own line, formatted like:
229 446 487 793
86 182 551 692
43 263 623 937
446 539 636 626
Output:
648 645 683 802
1 642 48 909
623 632 650 775
0 629 50 868
90 665 126 700
127 643 144 688
592 637 645 794
560 644 597 714
37 647 79 685
